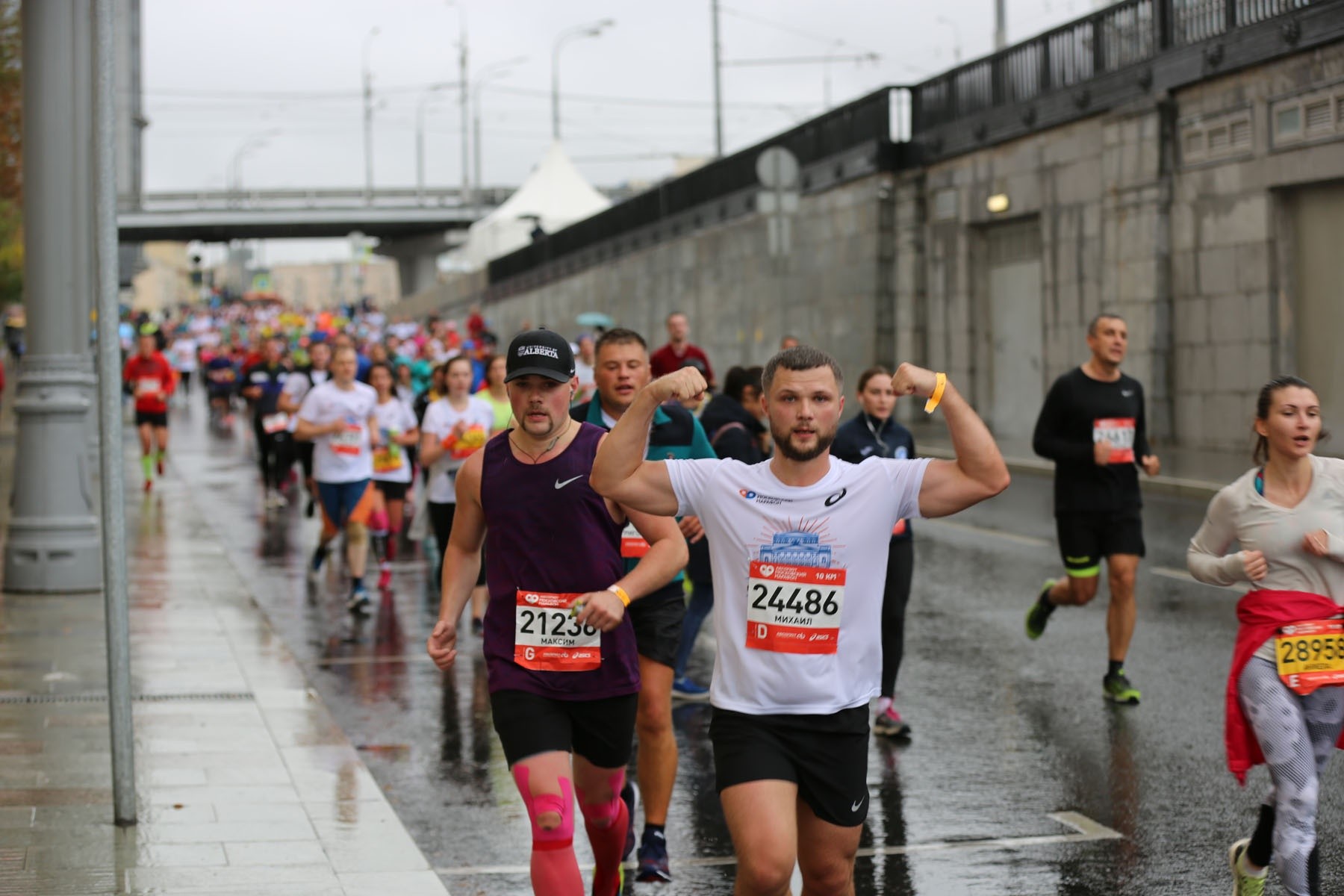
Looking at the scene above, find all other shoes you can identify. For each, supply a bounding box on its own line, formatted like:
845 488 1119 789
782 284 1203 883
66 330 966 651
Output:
310 547 324 575
156 459 164 473
471 617 485 636
143 479 151 490
263 492 287 508
386 530 397 561
671 675 711 699
345 586 370 610
378 569 391 590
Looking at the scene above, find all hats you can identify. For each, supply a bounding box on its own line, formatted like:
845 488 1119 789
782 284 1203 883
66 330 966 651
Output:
503 328 575 383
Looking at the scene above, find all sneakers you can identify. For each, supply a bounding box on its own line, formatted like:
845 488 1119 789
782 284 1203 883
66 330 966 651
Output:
1227 838 1268 896
874 707 912 737
637 828 670 879
1024 577 1058 640
1102 667 1141 704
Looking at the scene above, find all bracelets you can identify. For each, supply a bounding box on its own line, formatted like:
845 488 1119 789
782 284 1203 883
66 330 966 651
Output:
606 584 630 607
924 372 946 415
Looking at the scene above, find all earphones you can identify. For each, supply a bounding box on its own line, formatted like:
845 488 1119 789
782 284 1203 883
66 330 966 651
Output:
860 396 864 402
570 389 574 395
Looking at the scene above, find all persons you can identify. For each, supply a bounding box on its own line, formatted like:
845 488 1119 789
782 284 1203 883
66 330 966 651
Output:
589 345 1012 896
1187 375 1344 896
84 285 925 738
422 330 691 896
1023 312 1161 706
570 328 719 885
